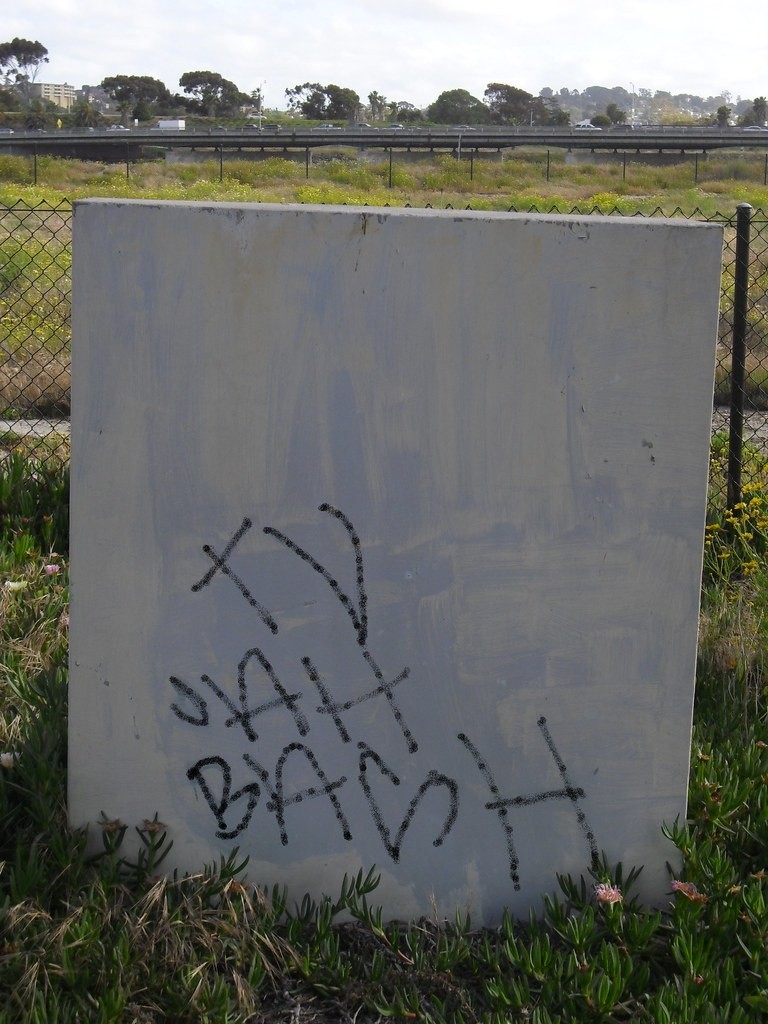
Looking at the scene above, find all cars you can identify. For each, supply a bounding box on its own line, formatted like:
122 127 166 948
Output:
2 118 606 133
744 125 761 130
